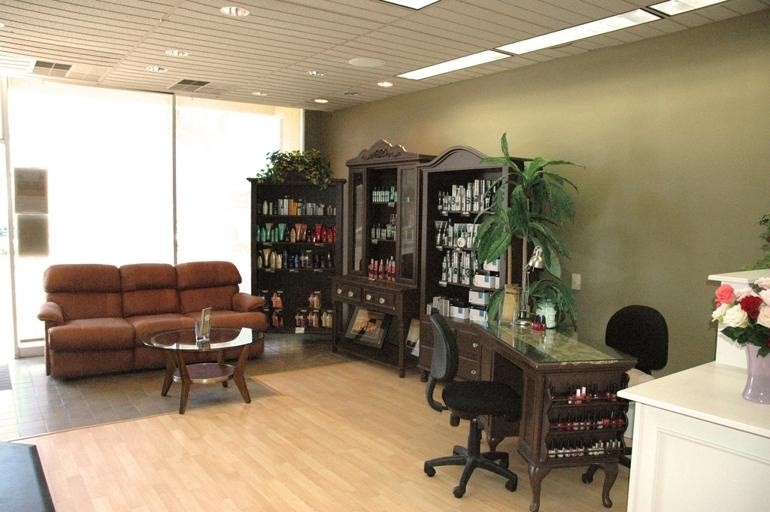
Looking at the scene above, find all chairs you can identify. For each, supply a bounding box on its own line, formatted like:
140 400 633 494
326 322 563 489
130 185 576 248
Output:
424 307 518 498
605 305 669 375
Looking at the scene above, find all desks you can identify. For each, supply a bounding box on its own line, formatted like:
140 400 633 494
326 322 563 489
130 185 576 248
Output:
471 321 637 512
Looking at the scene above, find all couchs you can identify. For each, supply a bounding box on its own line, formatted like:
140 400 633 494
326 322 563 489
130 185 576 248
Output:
38 258 266 381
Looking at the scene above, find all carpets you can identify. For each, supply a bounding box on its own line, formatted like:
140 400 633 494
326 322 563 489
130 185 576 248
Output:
0 357 283 443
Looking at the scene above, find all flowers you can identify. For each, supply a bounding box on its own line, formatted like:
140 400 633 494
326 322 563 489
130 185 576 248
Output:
711 278 770 357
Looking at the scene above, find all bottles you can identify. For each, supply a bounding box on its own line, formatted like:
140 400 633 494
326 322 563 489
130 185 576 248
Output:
262 195 332 215
295 290 333 329
381 184 385 202
371 184 376 203
261 289 285 329
376 184 381 202
269 249 331 267
368 256 395 273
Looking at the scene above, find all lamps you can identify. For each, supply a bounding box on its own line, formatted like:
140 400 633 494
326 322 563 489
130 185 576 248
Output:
529 245 560 281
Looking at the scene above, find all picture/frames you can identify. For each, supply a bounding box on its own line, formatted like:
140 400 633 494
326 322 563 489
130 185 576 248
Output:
404 318 421 349
345 307 390 348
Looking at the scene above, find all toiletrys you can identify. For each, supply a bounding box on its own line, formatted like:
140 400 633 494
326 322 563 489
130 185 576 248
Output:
547 384 623 460
368 184 397 279
259 194 333 327
437 179 498 285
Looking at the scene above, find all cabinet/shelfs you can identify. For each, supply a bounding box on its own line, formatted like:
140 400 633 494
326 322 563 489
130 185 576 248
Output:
247 178 347 341
619 268 770 512
421 145 530 389
331 140 436 379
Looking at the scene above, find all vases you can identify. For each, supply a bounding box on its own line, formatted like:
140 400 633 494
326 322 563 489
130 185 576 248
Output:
742 343 769 402
